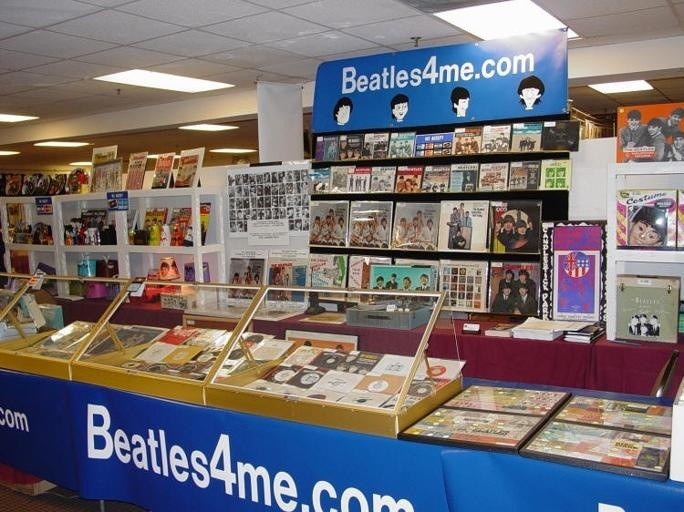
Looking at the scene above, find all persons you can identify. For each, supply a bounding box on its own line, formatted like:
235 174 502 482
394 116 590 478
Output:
333 97 353 126
629 314 659 336
628 206 666 246
146 219 183 277
518 75 545 112
391 94 409 122
451 86 470 118
619 108 684 162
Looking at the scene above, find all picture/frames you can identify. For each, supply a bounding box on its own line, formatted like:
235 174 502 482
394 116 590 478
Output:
396 381 573 455
519 391 670 485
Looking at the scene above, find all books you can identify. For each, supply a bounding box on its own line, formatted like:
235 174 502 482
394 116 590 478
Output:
484 316 606 344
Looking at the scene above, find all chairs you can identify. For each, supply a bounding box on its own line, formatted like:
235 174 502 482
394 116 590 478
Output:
652 346 680 404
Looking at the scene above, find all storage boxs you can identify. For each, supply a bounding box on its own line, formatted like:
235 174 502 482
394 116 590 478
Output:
344 298 434 333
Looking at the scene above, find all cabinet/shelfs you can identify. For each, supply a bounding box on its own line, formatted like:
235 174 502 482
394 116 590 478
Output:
52 189 226 313
70 278 268 406
603 159 684 345
1 273 137 381
204 285 463 437
0 192 61 302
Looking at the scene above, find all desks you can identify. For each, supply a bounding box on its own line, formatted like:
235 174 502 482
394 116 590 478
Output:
588 332 683 401
408 315 597 397
